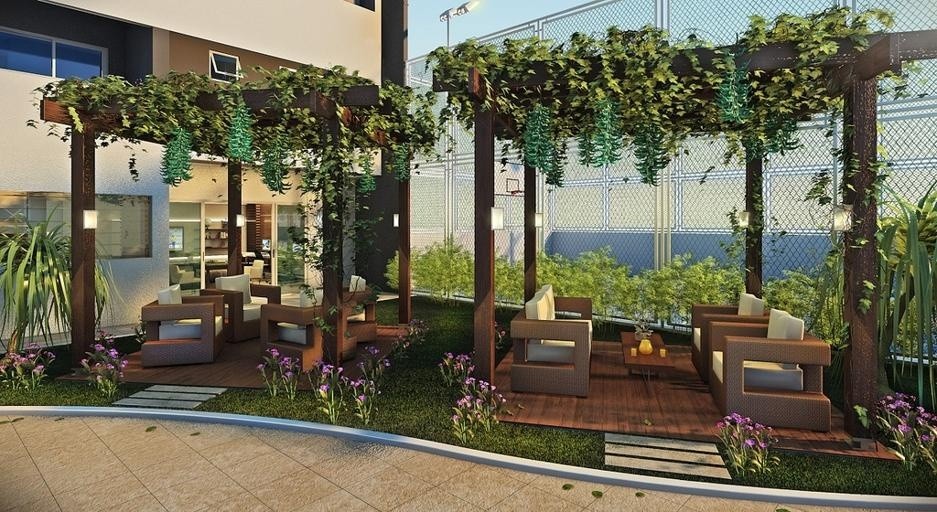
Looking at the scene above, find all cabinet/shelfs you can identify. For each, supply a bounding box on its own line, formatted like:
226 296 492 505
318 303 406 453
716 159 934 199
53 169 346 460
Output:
194 226 228 252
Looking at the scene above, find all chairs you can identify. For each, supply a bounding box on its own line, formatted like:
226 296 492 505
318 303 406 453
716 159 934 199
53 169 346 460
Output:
168 262 202 296
198 273 282 347
690 292 775 387
705 309 832 435
509 282 595 400
139 293 226 367
259 285 356 373
343 273 378 346
244 259 264 288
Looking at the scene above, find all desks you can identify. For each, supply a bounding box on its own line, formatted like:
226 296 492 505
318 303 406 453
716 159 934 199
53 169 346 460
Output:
192 261 229 284
621 326 674 394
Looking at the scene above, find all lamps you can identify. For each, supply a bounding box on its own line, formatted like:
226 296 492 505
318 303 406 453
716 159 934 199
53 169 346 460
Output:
737 212 750 231
236 213 246 227
535 212 546 230
393 214 399 228
81 207 97 231
316 208 324 229
490 205 505 232
831 202 854 233
